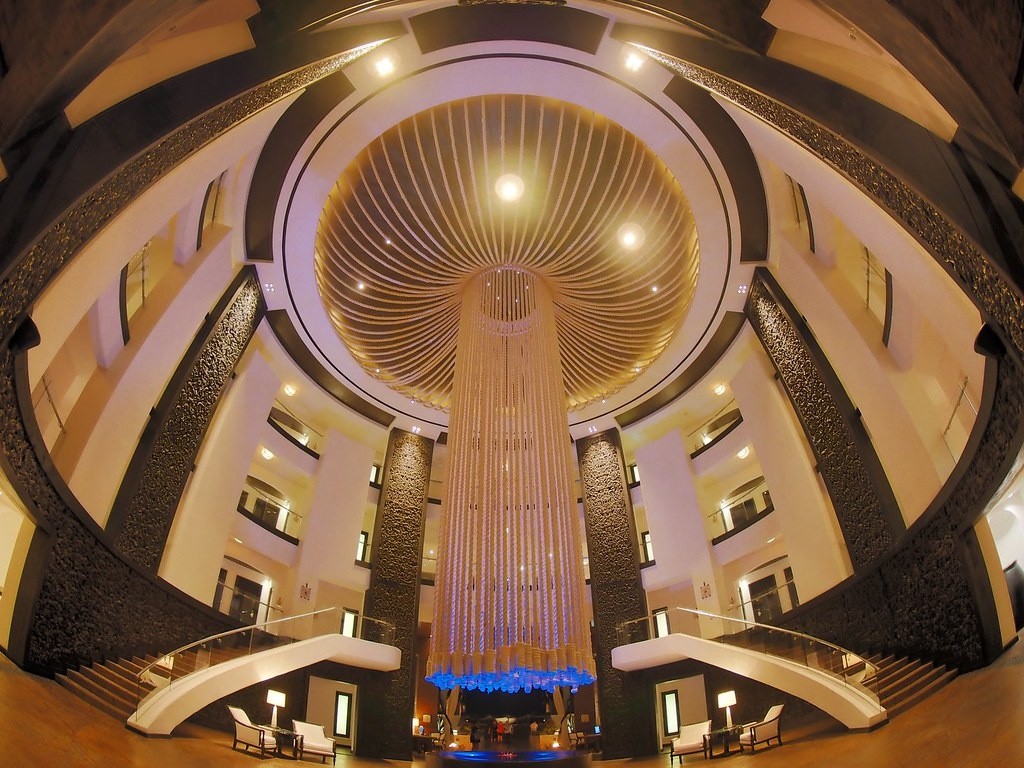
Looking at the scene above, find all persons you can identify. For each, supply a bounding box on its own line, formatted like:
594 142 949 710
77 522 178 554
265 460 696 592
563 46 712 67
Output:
488 720 514 744
470 726 481 751
530 721 539 736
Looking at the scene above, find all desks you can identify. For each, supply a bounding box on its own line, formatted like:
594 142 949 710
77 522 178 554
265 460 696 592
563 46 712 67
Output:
577 734 603 754
413 735 437 752
258 725 300 760
710 723 744 759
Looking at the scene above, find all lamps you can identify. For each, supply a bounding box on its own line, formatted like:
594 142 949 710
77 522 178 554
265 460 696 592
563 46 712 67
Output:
266 689 286 730
717 690 737 730
412 718 419 736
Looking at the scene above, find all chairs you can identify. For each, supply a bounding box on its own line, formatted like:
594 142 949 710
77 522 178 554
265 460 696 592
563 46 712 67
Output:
223 703 278 760
568 731 591 754
434 732 446 752
670 719 712 766
738 704 786 756
292 719 337 765
